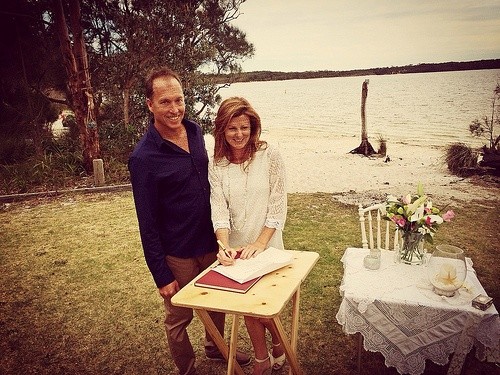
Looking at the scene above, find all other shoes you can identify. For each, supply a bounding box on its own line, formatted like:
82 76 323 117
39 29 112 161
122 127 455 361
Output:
206 349 251 366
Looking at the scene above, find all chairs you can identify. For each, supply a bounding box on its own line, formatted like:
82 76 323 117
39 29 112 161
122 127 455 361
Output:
357 200 404 251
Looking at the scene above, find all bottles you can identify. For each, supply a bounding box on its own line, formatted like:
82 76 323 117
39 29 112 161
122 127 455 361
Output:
426 244 467 297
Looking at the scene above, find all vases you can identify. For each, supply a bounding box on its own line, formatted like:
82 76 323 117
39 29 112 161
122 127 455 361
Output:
396 230 425 265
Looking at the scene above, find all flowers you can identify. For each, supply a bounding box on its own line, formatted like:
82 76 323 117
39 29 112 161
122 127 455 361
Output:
378 178 457 262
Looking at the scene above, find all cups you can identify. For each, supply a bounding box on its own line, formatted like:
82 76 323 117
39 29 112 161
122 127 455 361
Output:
364 248 381 270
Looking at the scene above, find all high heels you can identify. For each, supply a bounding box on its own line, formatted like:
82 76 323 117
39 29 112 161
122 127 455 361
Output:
252 352 272 375
272 342 286 370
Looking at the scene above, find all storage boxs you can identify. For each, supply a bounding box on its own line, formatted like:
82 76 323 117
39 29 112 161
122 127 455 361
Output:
471 293 494 311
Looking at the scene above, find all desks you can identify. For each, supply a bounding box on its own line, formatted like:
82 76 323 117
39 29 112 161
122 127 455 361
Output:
335 246 500 375
170 248 321 375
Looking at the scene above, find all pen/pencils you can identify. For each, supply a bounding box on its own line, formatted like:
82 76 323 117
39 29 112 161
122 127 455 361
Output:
217 239 236 264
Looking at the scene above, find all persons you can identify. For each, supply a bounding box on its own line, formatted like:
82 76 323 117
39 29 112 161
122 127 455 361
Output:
194 249 267 294
209 98 288 375
128 70 252 375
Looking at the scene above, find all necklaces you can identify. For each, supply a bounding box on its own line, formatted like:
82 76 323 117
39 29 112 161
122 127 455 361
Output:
227 156 249 232
